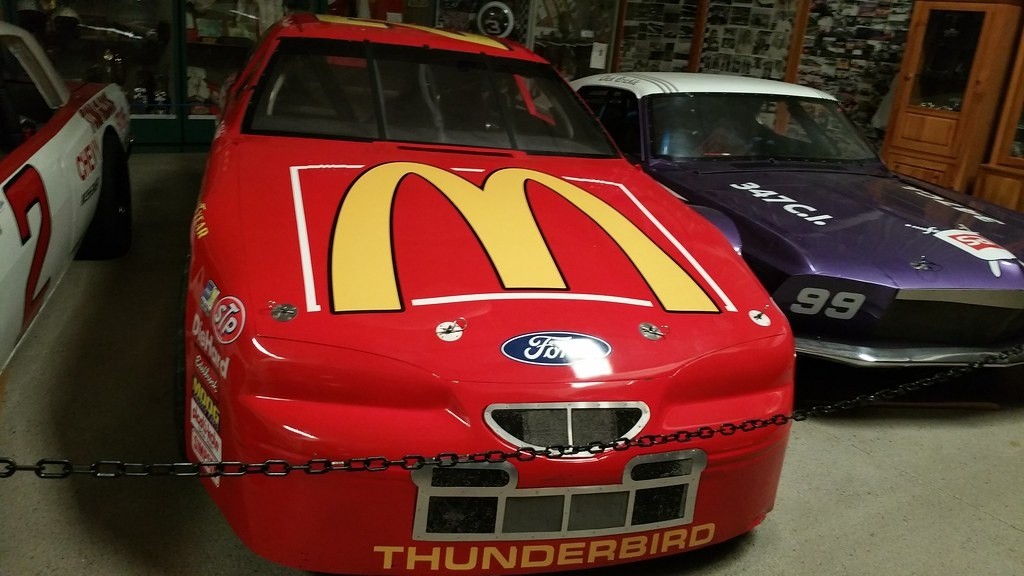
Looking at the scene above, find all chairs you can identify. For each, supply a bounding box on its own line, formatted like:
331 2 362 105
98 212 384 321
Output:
407 65 505 130
267 51 358 123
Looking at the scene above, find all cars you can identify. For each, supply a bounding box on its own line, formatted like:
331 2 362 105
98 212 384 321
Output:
0 21 137 377
178 16 794 576
518 71 1024 414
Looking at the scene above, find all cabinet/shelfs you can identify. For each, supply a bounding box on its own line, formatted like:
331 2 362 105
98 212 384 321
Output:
878 0 1024 214
42 0 327 152
